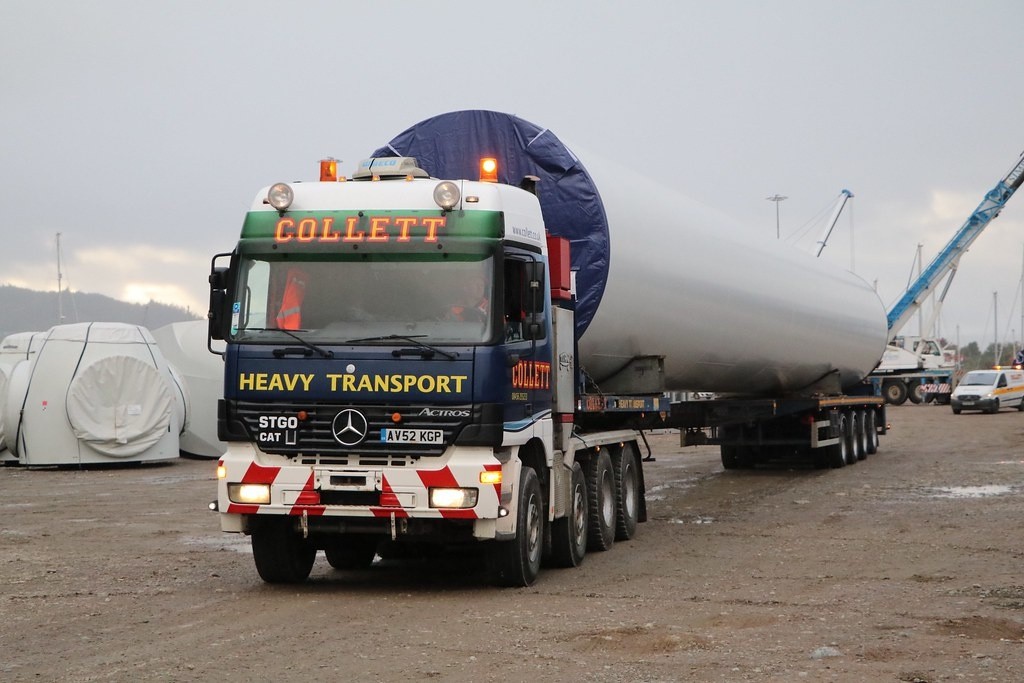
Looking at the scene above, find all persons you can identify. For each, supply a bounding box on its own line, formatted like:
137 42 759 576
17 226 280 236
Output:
442 276 488 324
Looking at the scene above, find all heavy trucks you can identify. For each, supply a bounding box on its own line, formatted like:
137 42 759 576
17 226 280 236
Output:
205 104 889 587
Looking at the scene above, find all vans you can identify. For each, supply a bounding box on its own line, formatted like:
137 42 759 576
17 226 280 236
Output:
950 366 1024 414
